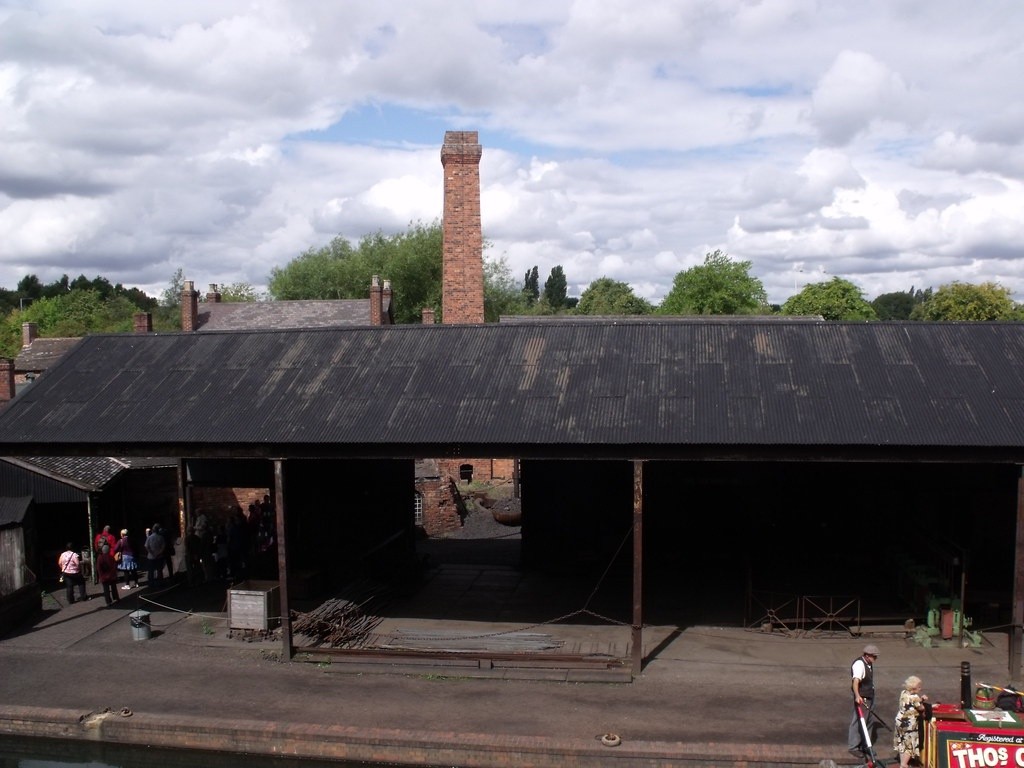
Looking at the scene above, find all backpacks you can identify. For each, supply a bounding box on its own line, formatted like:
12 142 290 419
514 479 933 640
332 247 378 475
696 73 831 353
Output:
97 533 110 553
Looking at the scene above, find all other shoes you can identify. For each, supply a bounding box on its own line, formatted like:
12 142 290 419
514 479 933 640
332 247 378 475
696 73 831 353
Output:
848 750 864 758
900 765 913 768
862 747 877 756
121 585 131 590
133 584 139 587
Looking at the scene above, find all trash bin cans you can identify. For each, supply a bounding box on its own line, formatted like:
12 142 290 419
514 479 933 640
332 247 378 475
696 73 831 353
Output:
129 610 151 642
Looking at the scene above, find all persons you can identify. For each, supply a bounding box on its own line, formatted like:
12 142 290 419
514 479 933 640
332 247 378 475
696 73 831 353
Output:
847 645 881 758
184 526 202 572
145 524 166 586
97 545 121 608
114 529 139 589
194 495 277 583
159 528 175 576
893 676 928 768
95 525 115 556
59 543 87 603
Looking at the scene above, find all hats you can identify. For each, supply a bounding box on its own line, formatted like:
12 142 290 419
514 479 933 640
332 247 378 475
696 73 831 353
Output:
186 527 197 532
863 644 880 656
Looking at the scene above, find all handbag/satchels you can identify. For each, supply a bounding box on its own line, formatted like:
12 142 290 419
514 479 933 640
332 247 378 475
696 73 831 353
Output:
56 574 64 585
918 701 932 720
114 551 121 562
